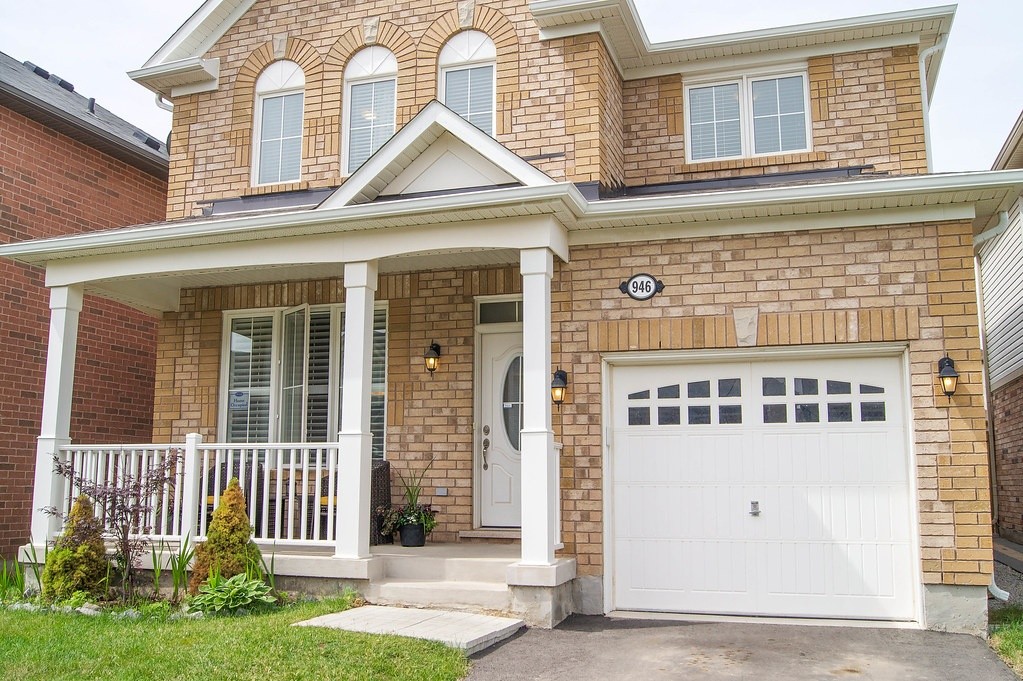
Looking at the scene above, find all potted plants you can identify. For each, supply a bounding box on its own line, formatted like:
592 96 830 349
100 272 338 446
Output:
379 455 440 547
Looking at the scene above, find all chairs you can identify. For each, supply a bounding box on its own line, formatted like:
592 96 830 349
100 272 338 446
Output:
309 460 394 546
197 460 262 539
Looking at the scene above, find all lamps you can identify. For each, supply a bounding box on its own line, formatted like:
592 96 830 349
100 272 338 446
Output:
551 366 568 412
936 352 960 404
423 337 441 378
